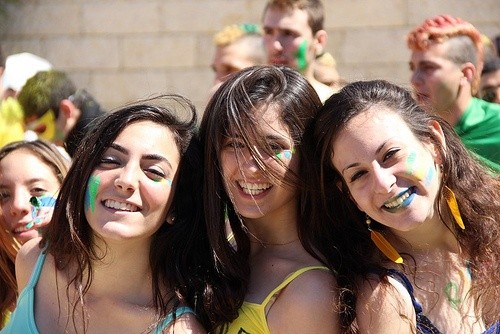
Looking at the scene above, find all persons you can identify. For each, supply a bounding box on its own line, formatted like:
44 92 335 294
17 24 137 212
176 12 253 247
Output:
258 0 339 105
0 0 500 334
